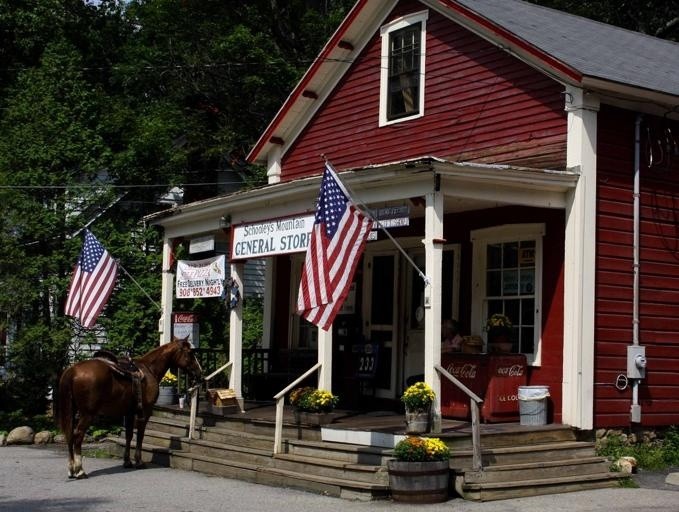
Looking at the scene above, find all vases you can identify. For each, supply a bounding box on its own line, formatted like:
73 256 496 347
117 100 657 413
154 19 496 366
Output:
403 411 431 435
293 410 336 428
385 457 449 506
155 385 174 408
492 331 516 355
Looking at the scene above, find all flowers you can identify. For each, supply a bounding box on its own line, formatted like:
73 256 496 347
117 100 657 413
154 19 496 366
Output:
400 379 437 410
157 369 179 387
486 310 513 335
286 384 342 413
391 434 451 462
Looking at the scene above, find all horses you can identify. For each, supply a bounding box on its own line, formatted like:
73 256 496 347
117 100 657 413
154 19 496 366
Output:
58 334 203 479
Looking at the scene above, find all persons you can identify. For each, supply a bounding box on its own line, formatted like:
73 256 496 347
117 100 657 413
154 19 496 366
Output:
438 319 464 352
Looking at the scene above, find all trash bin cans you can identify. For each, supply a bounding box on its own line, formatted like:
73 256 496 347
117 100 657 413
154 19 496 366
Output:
517 385 551 426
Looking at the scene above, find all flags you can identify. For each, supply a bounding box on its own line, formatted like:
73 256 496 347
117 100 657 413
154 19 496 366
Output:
293 166 375 331
62 229 119 329
